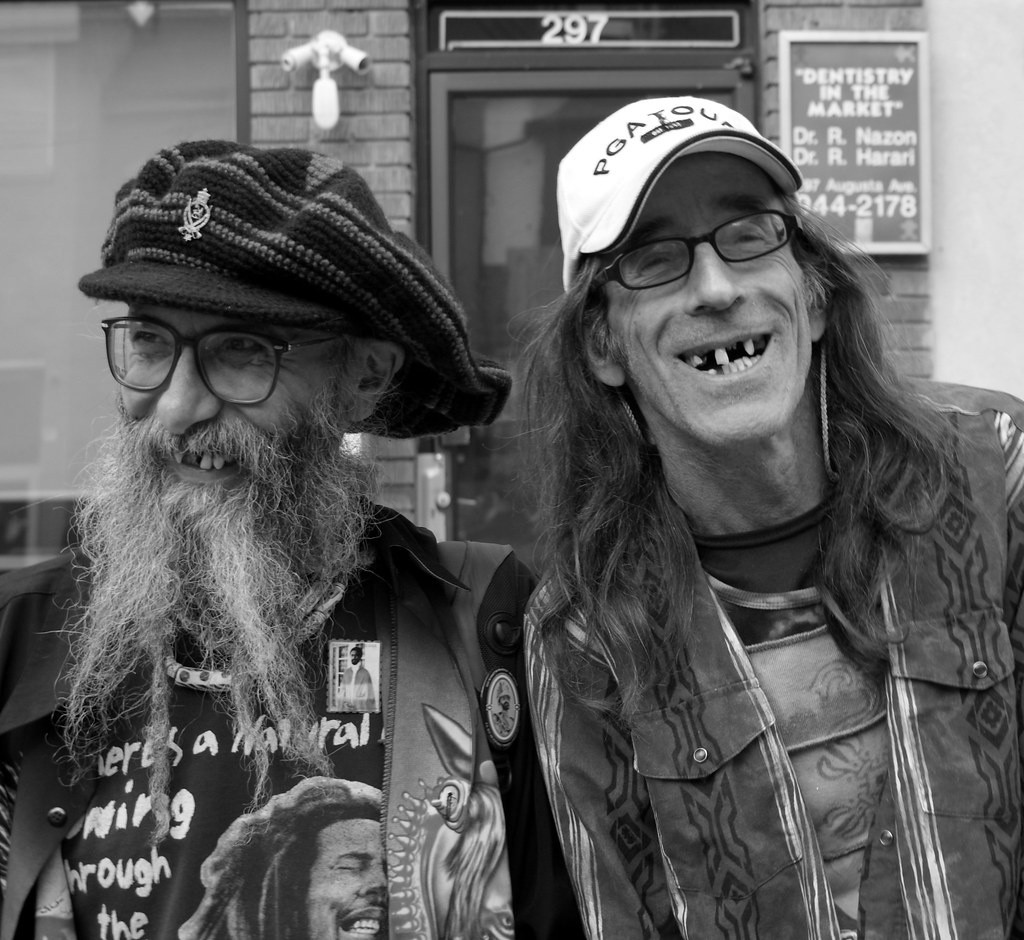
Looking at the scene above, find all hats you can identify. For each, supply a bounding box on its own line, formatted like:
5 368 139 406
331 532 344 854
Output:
556 95 802 294
77 141 512 439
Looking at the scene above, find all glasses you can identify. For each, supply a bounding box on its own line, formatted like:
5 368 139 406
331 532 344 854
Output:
592 211 794 290
99 316 340 406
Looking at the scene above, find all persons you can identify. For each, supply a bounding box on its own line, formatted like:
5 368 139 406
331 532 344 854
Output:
522 94 1024 940
0 143 587 940
338 646 377 711
177 777 388 940
